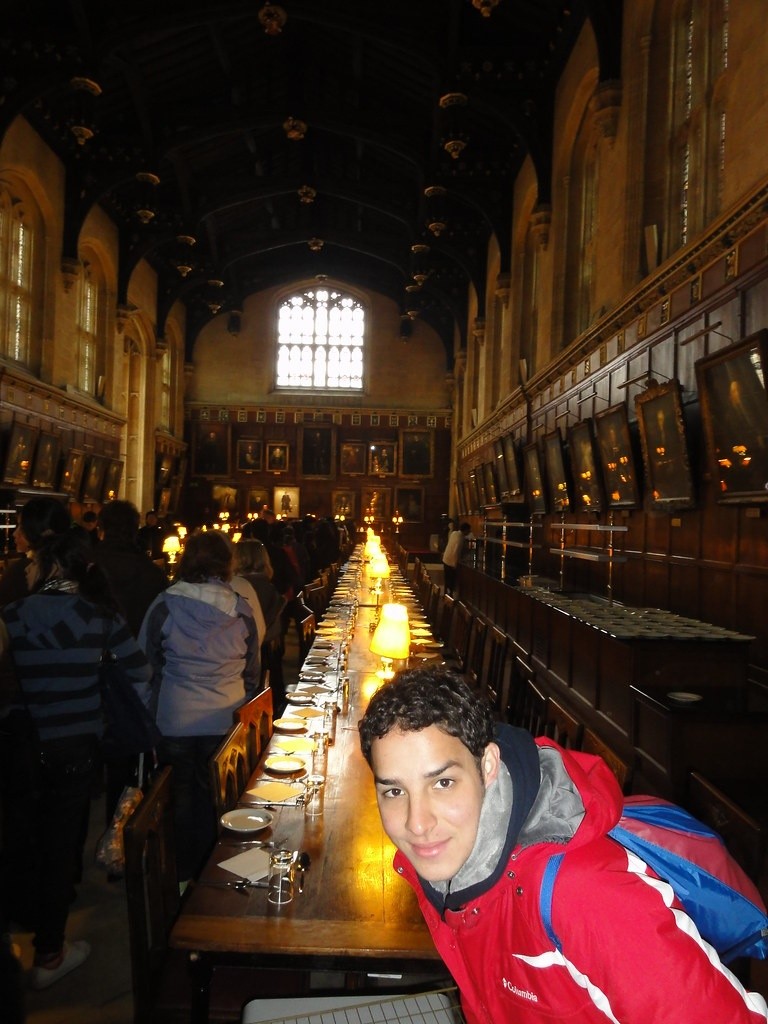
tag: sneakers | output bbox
[12,943,21,958]
[32,939,90,990]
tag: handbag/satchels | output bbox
[99,607,152,766]
[94,752,154,875]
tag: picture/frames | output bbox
[295,421,338,482]
[265,443,289,473]
[4,421,125,505]
[368,440,398,475]
[332,489,356,519]
[451,329,768,517]
[190,420,234,479]
[398,427,436,479]
[361,487,390,523]
[340,443,367,475]
[391,485,427,523]
[236,439,264,473]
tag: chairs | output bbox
[393,540,762,987]
[120,563,339,1024]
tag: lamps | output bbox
[162,513,413,686]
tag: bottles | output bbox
[273,718,307,730]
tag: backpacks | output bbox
[539,794,768,966]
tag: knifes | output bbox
[231,881,269,888]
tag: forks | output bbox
[219,838,288,848]
[200,881,251,889]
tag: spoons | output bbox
[298,852,310,894]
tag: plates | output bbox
[299,673,322,681]
[284,540,445,664]
[514,586,758,640]
[285,693,315,703]
[667,692,702,702]
[220,809,274,833]
[264,757,306,773]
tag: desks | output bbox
[168,538,452,962]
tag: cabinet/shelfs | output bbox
[549,513,630,610]
[483,514,544,584]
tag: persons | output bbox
[357,665,768,1024]
[440,518,471,596]
[0,497,353,1024]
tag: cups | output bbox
[268,542,416,905]
